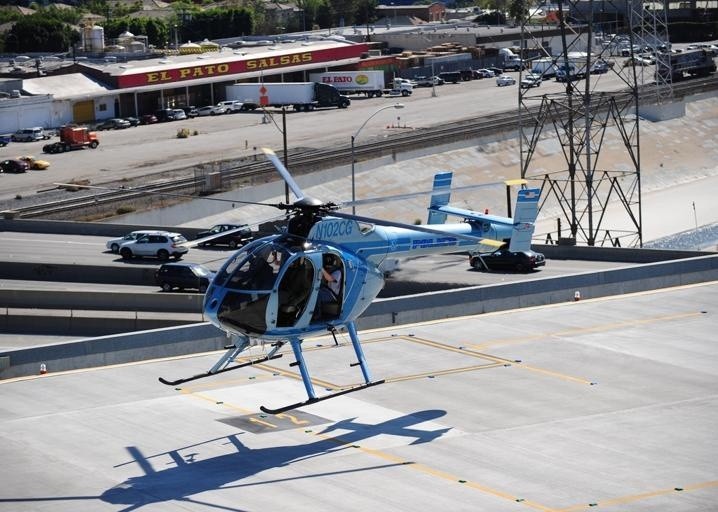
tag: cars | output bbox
[98,100,245,131]
[521,75,542,88]
[496,76,516,86]
[406,65,504,86]
[106,228,188,259]
[156,262,215,291]
[196,221,249,247]
[470,248,545,271]
[0,154,50,174]
[601,32,718,65]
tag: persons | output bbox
[296,257,343,319]
[230,249,274,297]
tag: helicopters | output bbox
[55,148,539,416]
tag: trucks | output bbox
[225,82,351,111]
[309,69,413,97]
[653,50,716,80]
[530,51,611,80]
[43,125,99,154]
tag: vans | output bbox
[0,126,44,146]
